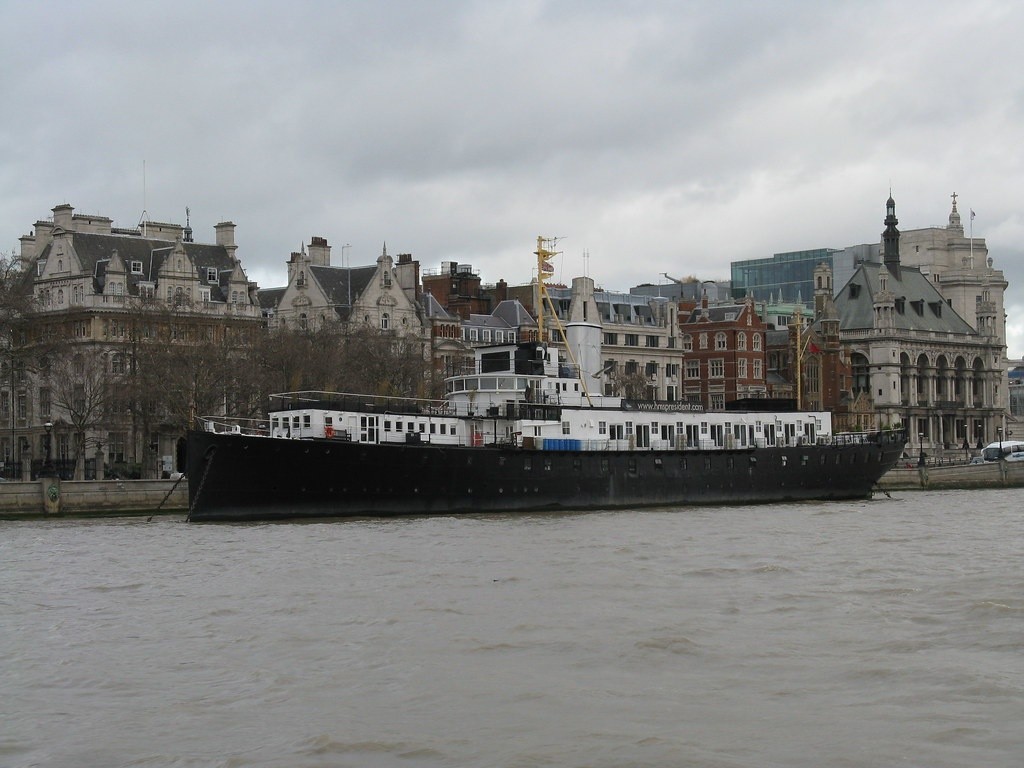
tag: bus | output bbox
[981,441,1024,464]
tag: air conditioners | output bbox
[626,434,637,450]
[818,437,830,446]
[798,435,808,447]
[776,438,786,448]
[676,435,686,450]
[724,434,735,450]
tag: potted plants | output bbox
[467,389,476,416]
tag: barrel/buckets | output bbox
[523,436,582,451]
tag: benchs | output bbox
[326,429,352,442]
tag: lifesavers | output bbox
[325,426,334,438]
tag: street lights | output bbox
[919,430,925,452]
[976,424,984,449]
[998,426,1006,459]
[43,419,55,478]
[962,422,970,449]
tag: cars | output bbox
[970,457,984,465]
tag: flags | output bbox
[808,340,820,352]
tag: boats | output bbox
[175,235,911,524]
